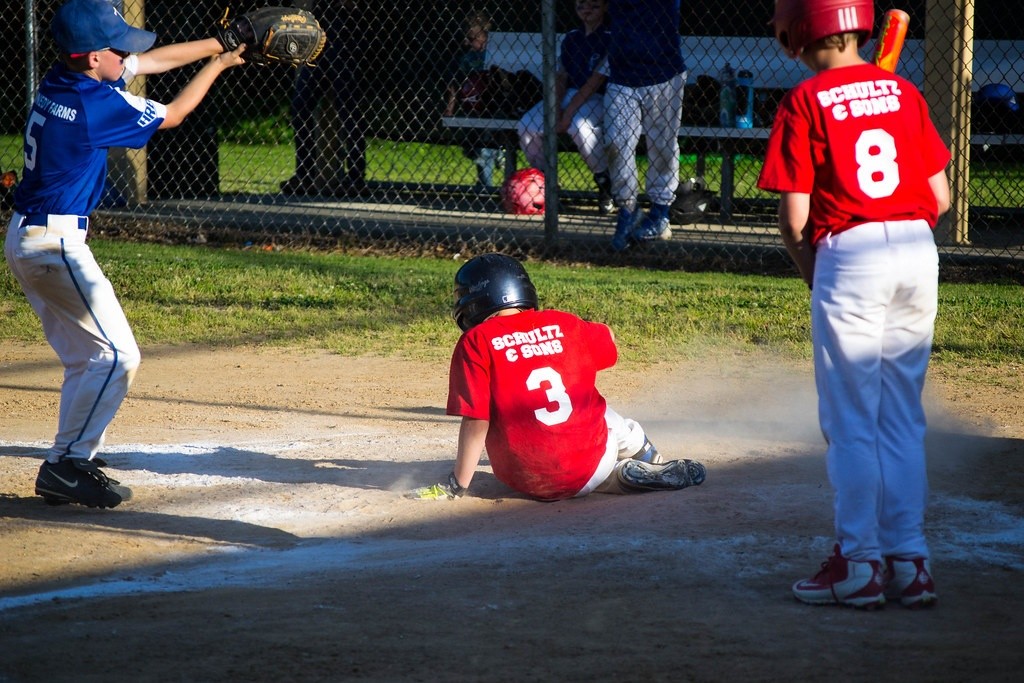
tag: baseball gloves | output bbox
[214,6,328,66]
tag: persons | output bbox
[754,0,950,607]
[3,0,326,509]
[402,252,705,503]
[433,1,687,252]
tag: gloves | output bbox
[403,473,466,502]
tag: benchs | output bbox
[441,31,1024,226]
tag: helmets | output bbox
[452,253,538,332]
[768,0,875,59]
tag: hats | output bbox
[51,0,157,58]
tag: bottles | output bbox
[718,62,754,128]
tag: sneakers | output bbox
[793,550,884,613]
[612,210,638,250]
[36,459,134,509]
[594,173,614,214]
[618,459,706,490]
[632,215,673,243]
[884,553,938,611]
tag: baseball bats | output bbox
[875,7,912,73]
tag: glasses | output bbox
[110,47,129,59]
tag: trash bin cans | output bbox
[973,84,1021,162]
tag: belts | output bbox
[21,215,88,230]
